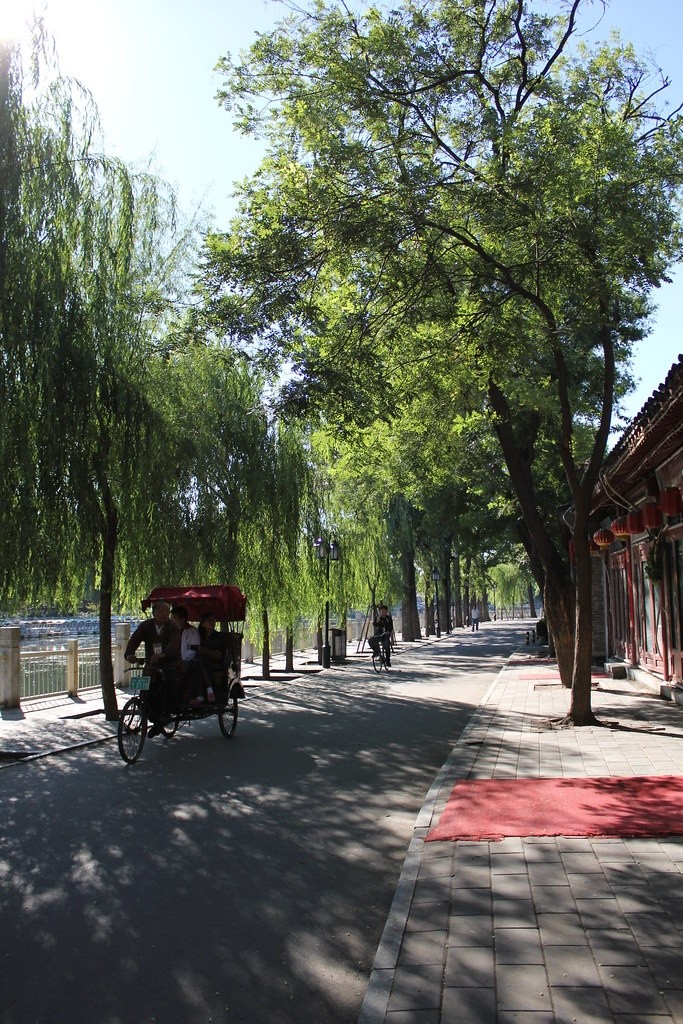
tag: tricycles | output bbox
[117,585,247,763]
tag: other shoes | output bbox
[208,692,215,702]
[386,659,391,667]
[148,726,161,739]
[190,698,205,705]
[157,709,169,720]
[372,651,381,657]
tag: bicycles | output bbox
[369,641,391,673]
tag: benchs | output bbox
[214,632,242,689]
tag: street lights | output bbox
[313,531,340,668]
[431,564,441,637]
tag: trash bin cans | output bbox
[328,628,347,662]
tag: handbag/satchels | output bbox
[183,653,214,683]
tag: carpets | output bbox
[425,775,683,842]
[507,660,556,665]
[519,672,610,680]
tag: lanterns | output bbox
[661,486,682,517]
[587,538,598,556]
[593,529,614,550]
[642,502,661,528]
[611,518,630,541]
[626,513,644,534]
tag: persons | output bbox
[471,605,479,632]
[368,605,393,667]
[125,603,227,739]
